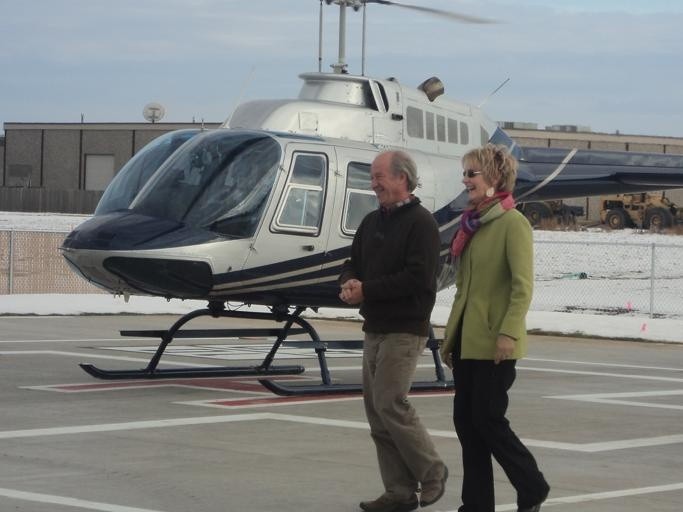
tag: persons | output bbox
[440,141,551,511]
[337,149,449,511]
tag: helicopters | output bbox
[57,0,683,400]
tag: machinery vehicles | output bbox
[512,198,586,229]
[598,190,683,232]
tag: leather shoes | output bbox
[361,494,418,511]
[421,467,448,506]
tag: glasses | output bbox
[463,169,483,178]
